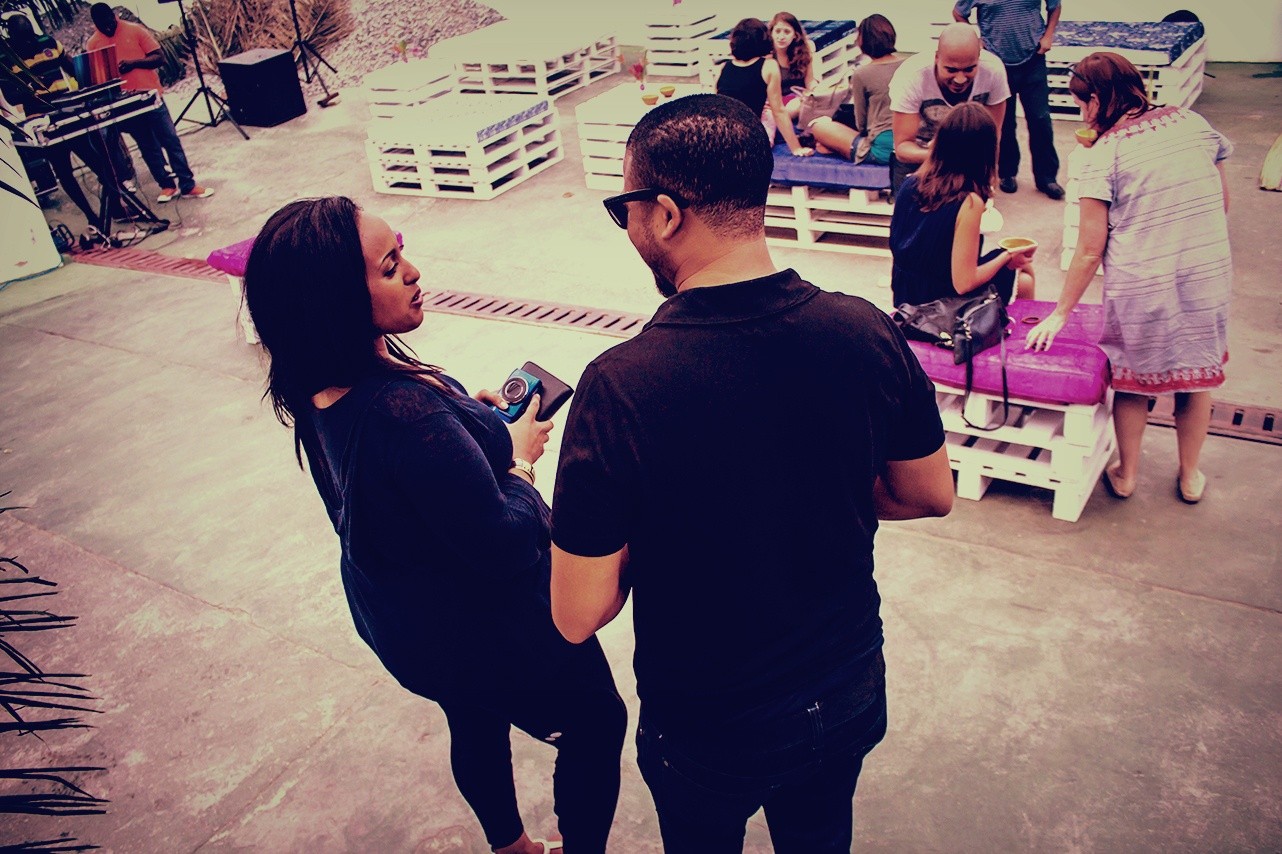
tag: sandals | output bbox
[533,838,562,854]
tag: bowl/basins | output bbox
[997,238,1038,258]
[660,86,675,97]
[641,95,658,105]
[1074,128,1098,147]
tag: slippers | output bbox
[1103,458,1134,499]
[1179,467,1206,503]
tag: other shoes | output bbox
[182,187,215,199]
[87,226,100,238]
[1000,177,1018,193]
[123,179,136,192]
[157,187,180,202]
[114,206,139,222]
[39,199,59,209]
[1036,180,1065,198]
[816,142,834,154]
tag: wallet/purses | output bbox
[490,359,573,424]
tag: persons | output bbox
[811,14,907,165]
[651,0,1232,506]
[87,5,214,202]
[236,192,627,854]
[0,14,139,232]
[551,95,958,854]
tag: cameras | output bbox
[489,367,541,423]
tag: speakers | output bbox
[217,49,306,127]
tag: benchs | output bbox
[700,19,862,96]
[367,92,566,200]
[763,121,898,256]
[1017,21,1204,121]
[429,17,621,99]
[887,300,1117,523]
[207,232,403,345]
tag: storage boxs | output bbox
[10,89,163,147]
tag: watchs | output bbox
[509,458,536,485]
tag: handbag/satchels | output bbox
[789,83,853,131]
[893,292,1013,366]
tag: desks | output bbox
[1061,143,1107,274]
[366,57,460,137]
[576,81,700,190]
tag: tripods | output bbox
[158,0,250,140]
[287,0,337,95]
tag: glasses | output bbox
[1065,64,1096,93]
[603,185,690,230]
